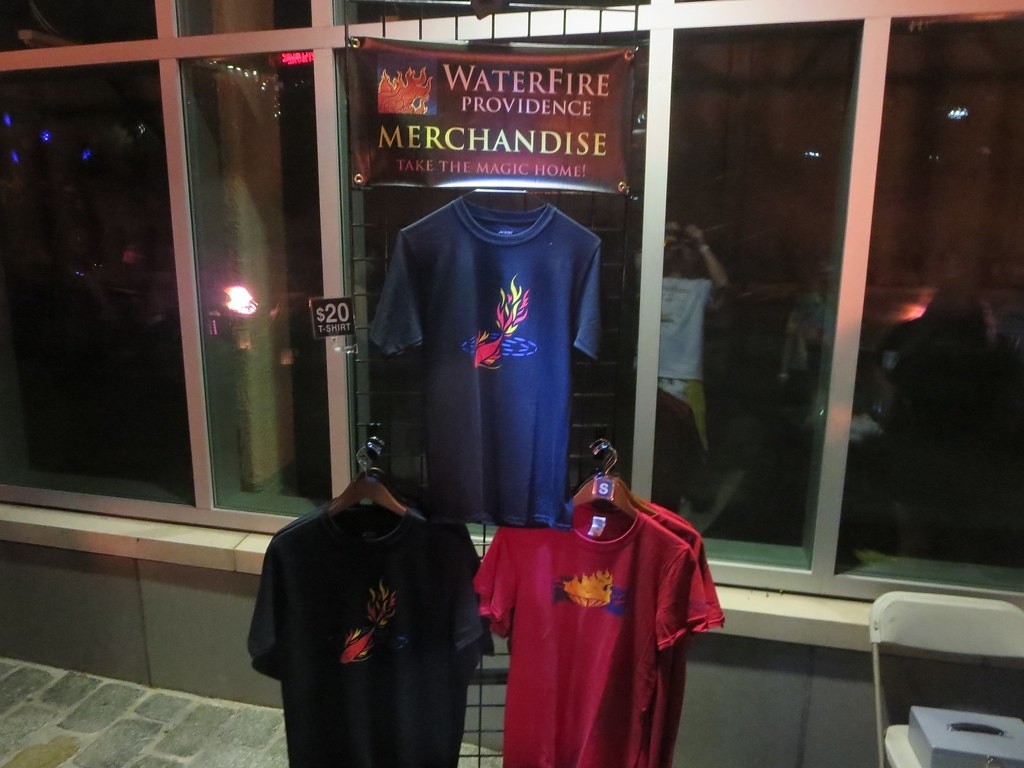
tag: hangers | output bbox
[463,189,547,211]
[329,435,406,520]
[572,439,658,523]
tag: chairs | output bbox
[869,591,1024,768]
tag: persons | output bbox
[796,267,999,446]
[624,223,727,451]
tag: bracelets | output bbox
[700,245,709,252]
